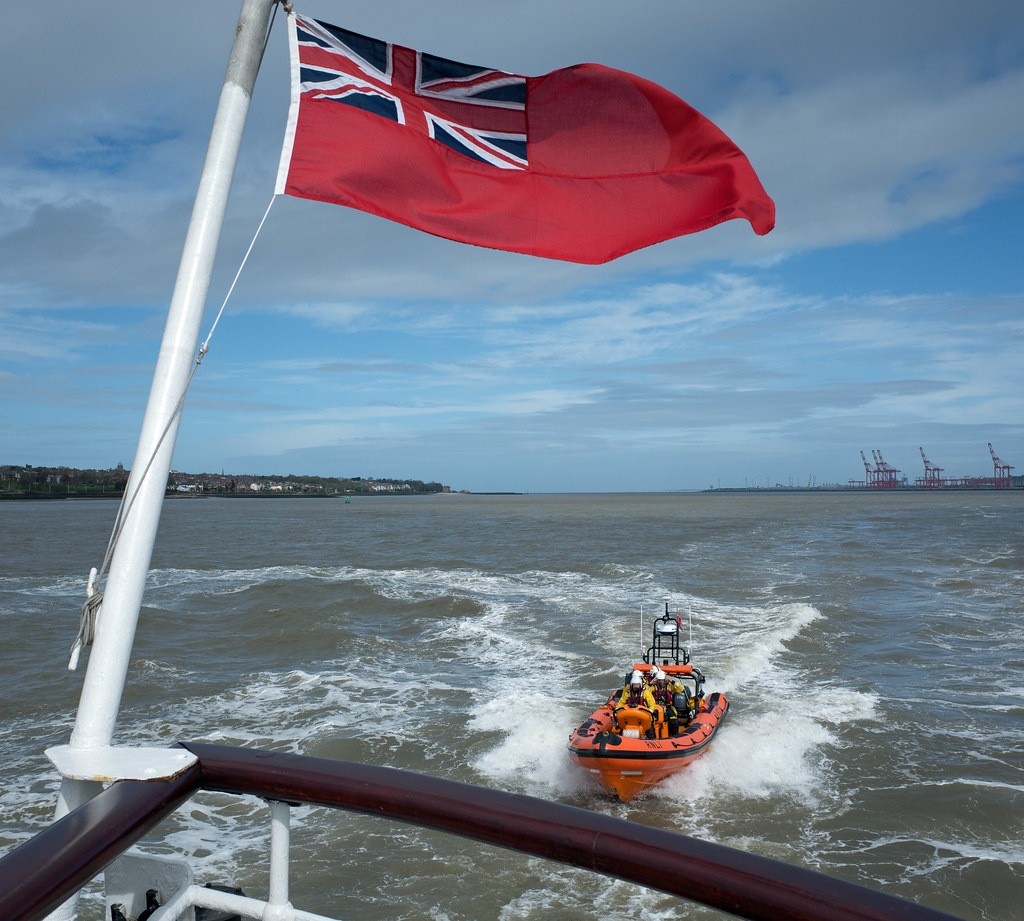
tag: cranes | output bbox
[919,446,944,487]
[860,449,901,489]
[988,442,1015,487]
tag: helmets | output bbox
[656,671,666,679]
[631,676,643,688]
[650,666,661,677]
[632,670,644,678]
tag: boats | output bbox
[565,595,730,804]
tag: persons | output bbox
[614,676,655,740]
[632,665,662,693]
[647,671,684,716]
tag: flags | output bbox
[271,12,775,264]
[677,610,682,630]
